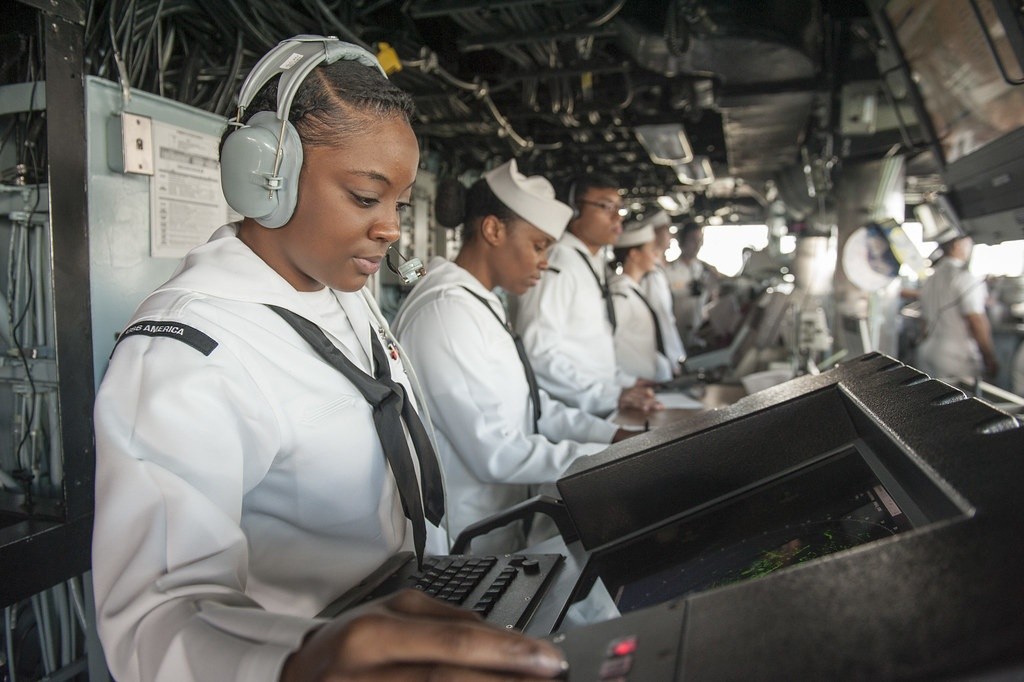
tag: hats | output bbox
[927,247,946,267]
[483,157,574,243]
[610,220,655,248]
[638,207,671,229]
[935,224,973,245]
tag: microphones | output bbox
[383,245,427,284]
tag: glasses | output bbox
[574,196,628,216]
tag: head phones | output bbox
[568,173,583,219]
[222,32,388,228]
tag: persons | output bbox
[515,171,663,416]
[913,217,1000,383]
[91,34,570,682]
[390,157,649,555]
[609,198,721,384]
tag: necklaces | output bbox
[376,325,400,361]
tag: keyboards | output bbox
[303,549,564,630]
